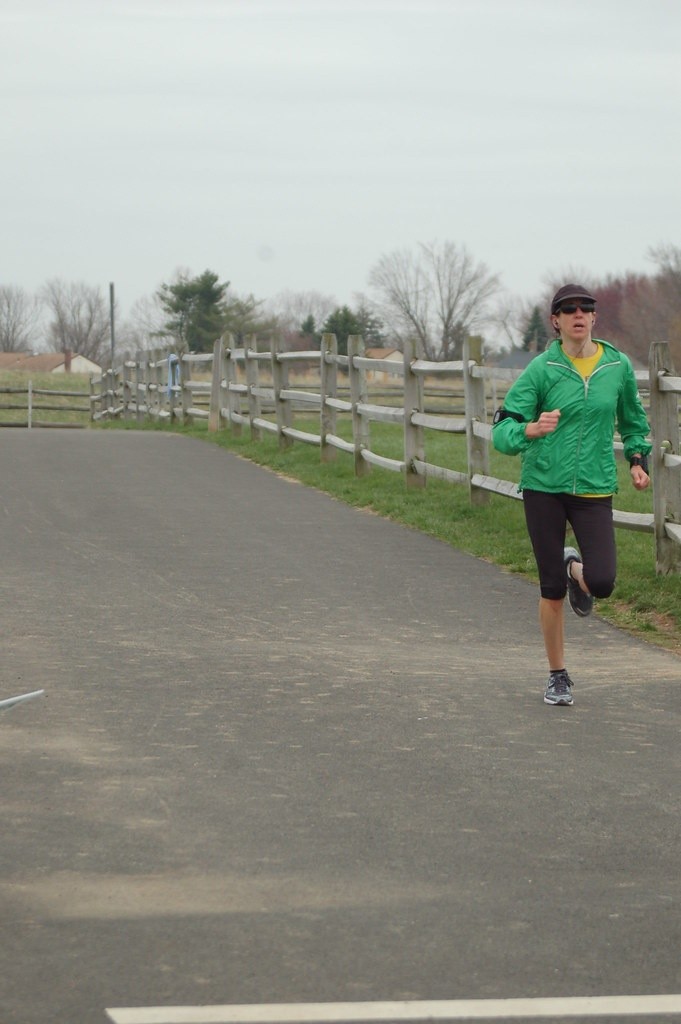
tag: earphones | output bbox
[592,320,594,324]
[554,320,558,325]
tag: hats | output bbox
[551,284,597,313]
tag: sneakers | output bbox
[544,670,573,706]
[564,547,593,617]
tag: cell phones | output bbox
[643,455,649,474]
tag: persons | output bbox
[492,281,652,707]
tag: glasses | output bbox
[552,302,594,314]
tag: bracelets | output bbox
[629,455,644,469]
